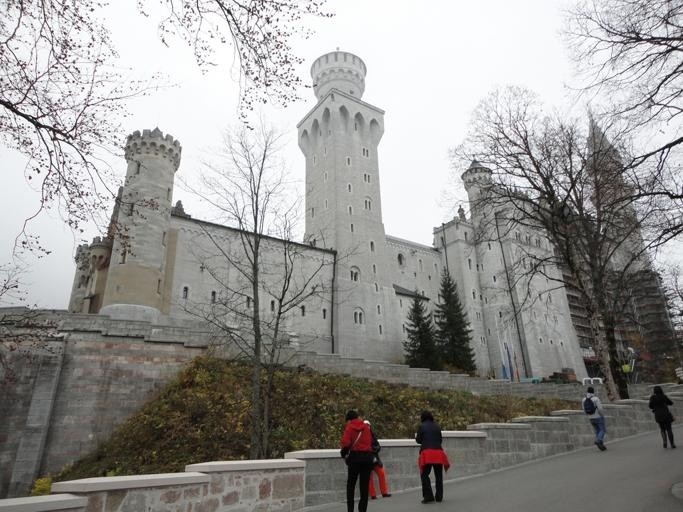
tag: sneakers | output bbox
[421,498,443,504]
[381,494,391,498]
[371,495,378,500]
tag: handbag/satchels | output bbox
[340,447,352,461]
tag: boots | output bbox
[661,432,676,449]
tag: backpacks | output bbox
[584,395,597,415]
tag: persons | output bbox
[626,346,640,364]
[362,420,392,500]
[649,385,676,450]
[338,409,375,512]
[415,409,451,503]
[581,386,609,452]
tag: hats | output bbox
[363,419,371,426]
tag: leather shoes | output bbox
[594,440,607,451]
